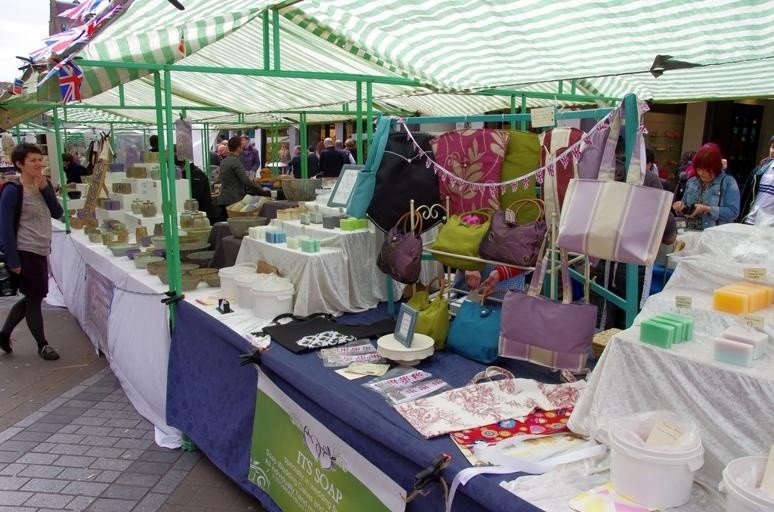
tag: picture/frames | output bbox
[327,164,365,208]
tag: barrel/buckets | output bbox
[218,262,258,296]
[251,281,297,319]
[234,273,271,308]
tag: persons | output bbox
[174,134,357,226]
[0,141,64,360]
[739,134,774,229]
[149,135,159,151]
[611,134,741,243]
[59,141,97,199]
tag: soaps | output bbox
[711,281,774,315]
[714,327,770,367]
[639,312,695,346]
[248,206,369,252]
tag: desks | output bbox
[161,287,774,512]
[45,214,217,453]
[234,197,442,316]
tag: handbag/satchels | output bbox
[0,262,17,297]
[429,206,490,275]
[496,285,600,373]
[444,288,509,368]
[369,210,428,285]
[403,274,448,351]
[483,198,544,269]
[552,93,678,269]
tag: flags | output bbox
[56,58,84,104]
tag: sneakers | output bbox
[36,341,61,361]
[0,330,14,354]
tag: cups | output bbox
[66,148,181,243]
[270,191,277,201]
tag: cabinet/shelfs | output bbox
[568,224,774,486]
[95,176,189,240]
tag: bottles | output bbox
[180,198,210,229]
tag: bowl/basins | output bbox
[280,178,324,202]
[106,236,219,289]
[228,216,266,238]
[226,197,274,218]
[217,265,294,319]
